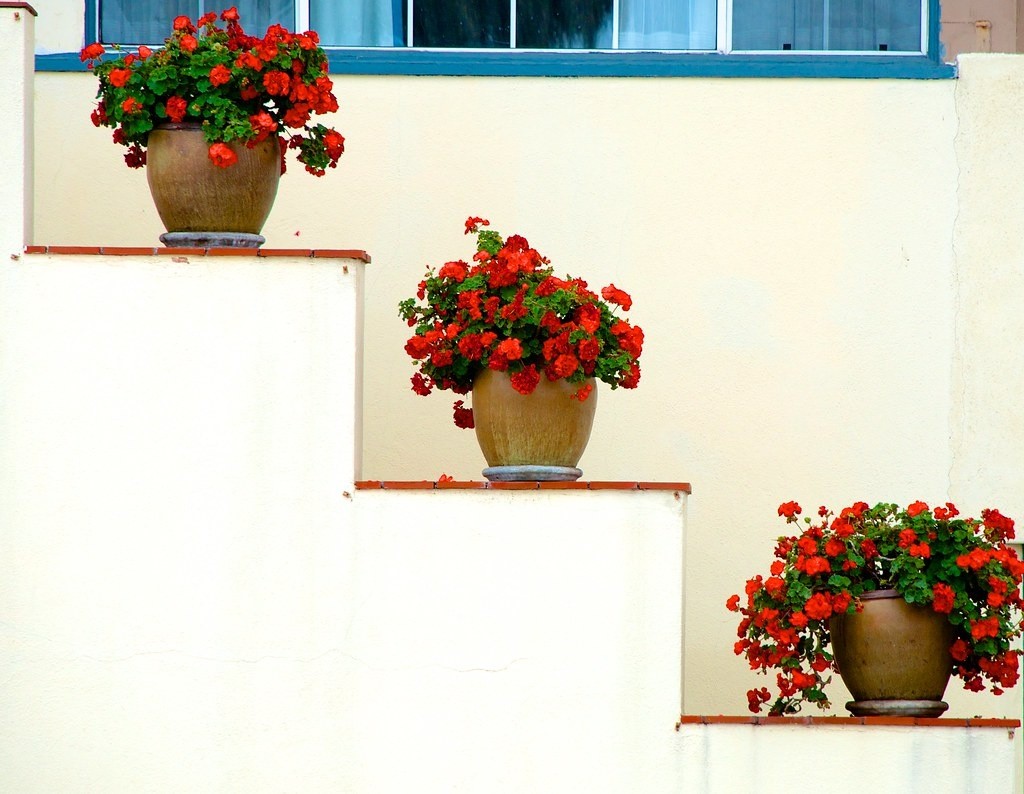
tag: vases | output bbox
[147,114,281,248]
[471,362,598,481]
[829,589,958,717]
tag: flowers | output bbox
[724,500,1024,717]
[78,7,345,178]
[397,214,645,430]
